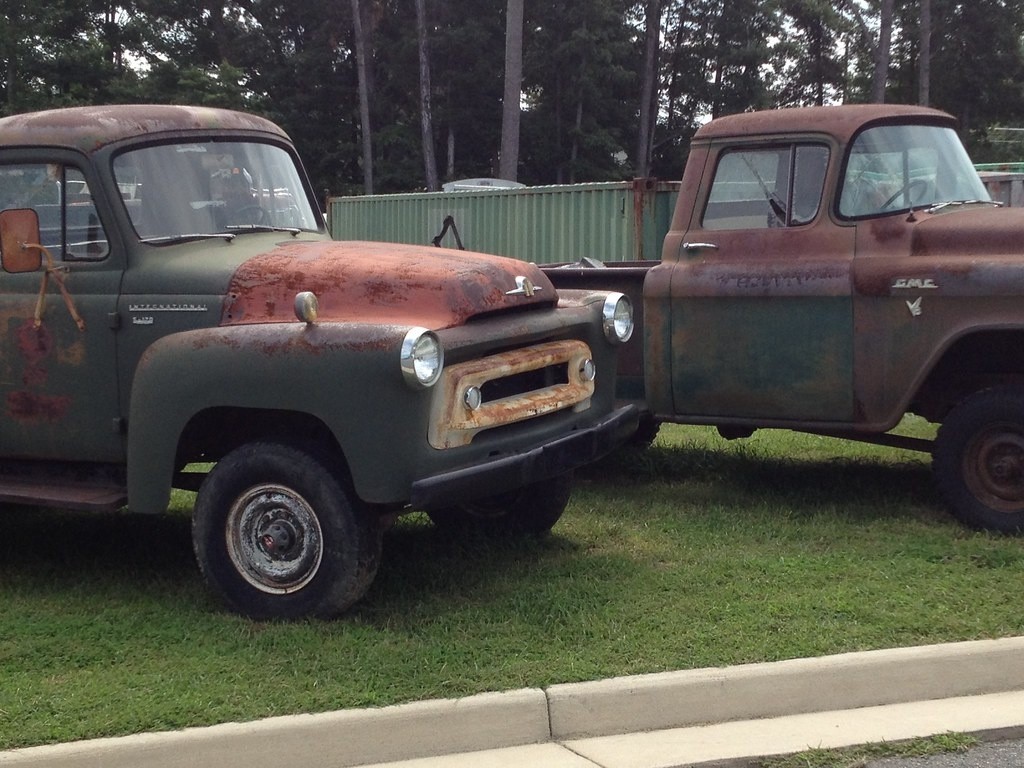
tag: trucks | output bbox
[0,105,637,622]
[324,102,1024,537]
